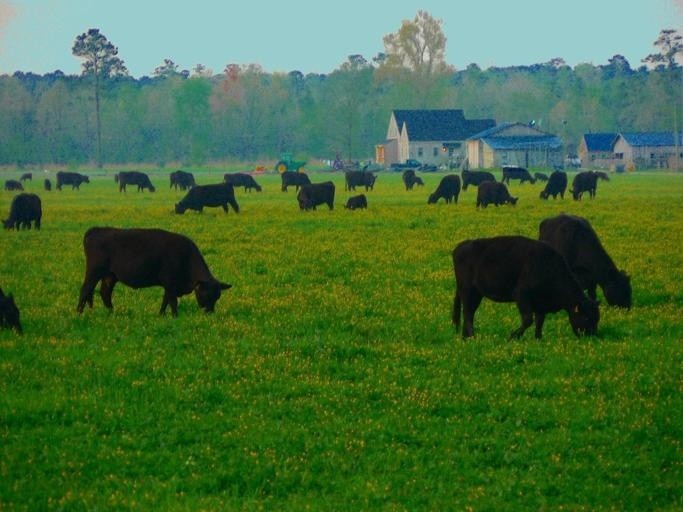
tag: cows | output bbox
[534,172,549,182]
[0,193,43,232]
[501,166,537,186]
[223,173,262,194]
[77,226,232,320]
[176,170,197,191]
[569,170,597,201]
[5,180,25,192]
[594,171,610,181]
[476,181,519,209]
[538,212,632,315]
[174,182,240,215]
[56,171,90,192]
[461,169,497,191]
[451,234,603,343]
[539,170,568,200]
[402,169,424,191]
[45,179,52,190]
[345,171,378,192]
[297,181,336,211]
[119,171,156,193]
[427,174,461,205]
[343,194,368,210]
[0,286,24,340]
[114,174,118,183]
[281,171,311,193]
[169,172,190,191]
[19,172,32,183]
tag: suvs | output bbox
[566,155,581,168]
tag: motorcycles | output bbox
[418,164,437,172]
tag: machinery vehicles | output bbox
[256,153,307,174]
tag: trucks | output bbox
[391,159,423,173]
